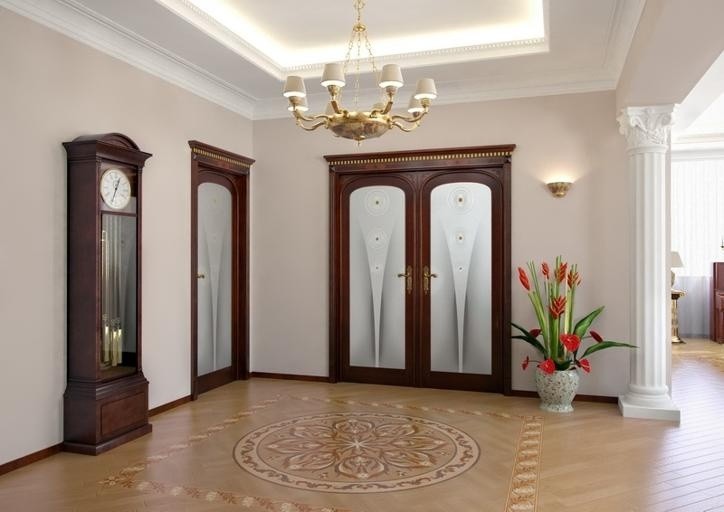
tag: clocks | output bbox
[56,129,153,456]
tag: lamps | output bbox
[669,251,683,292]
[282,0,438,141]
[546,181,574,199]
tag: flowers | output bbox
[510,255,640,374]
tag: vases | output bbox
[535,364,581,414]
